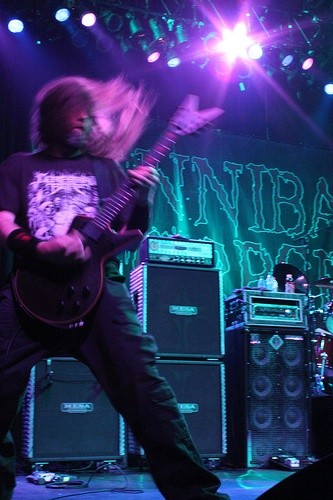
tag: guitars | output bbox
[12,95,224,325]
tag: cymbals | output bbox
[273,262,309,295]
[314,277,333,287]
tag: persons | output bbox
[0,78,234,500]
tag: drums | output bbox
[312,332,333,377]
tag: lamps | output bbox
[72,0,321,72]
